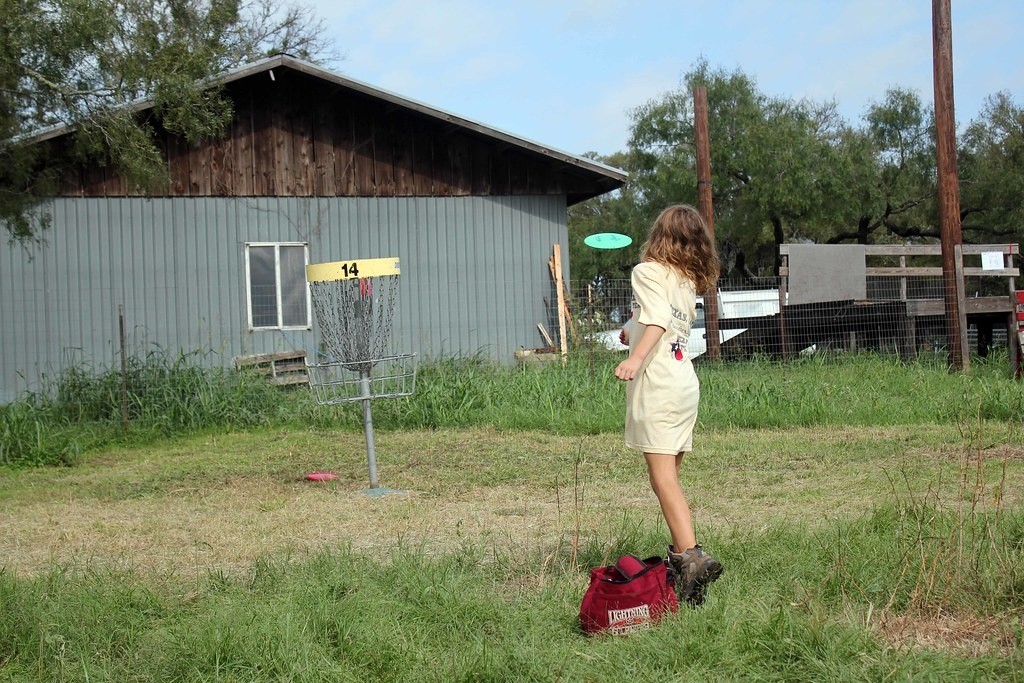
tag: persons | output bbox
[614,203,724,609]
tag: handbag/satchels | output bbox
[580,554,679,638]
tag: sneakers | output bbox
[664,556,681,584]
[667,545,723,610]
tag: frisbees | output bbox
[584,233,634,249]
